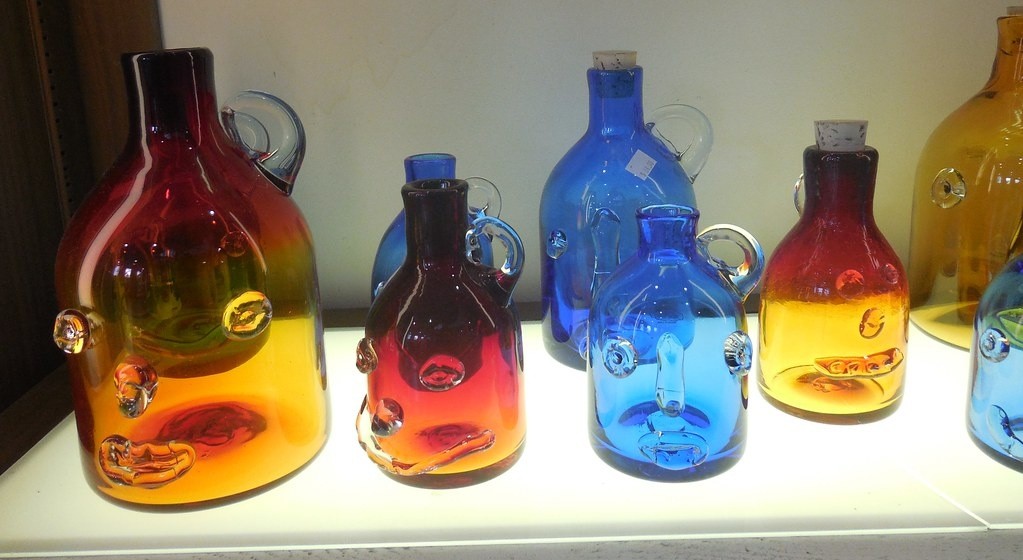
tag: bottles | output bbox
[371,153,502,305]
[908,7,1023,350]
[759,121,910,414]
[355,179,525,476]
[52,48,333,505]
[541,51,714,360]
[969,254,1023,463]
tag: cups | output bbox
[588,205,765,470]
